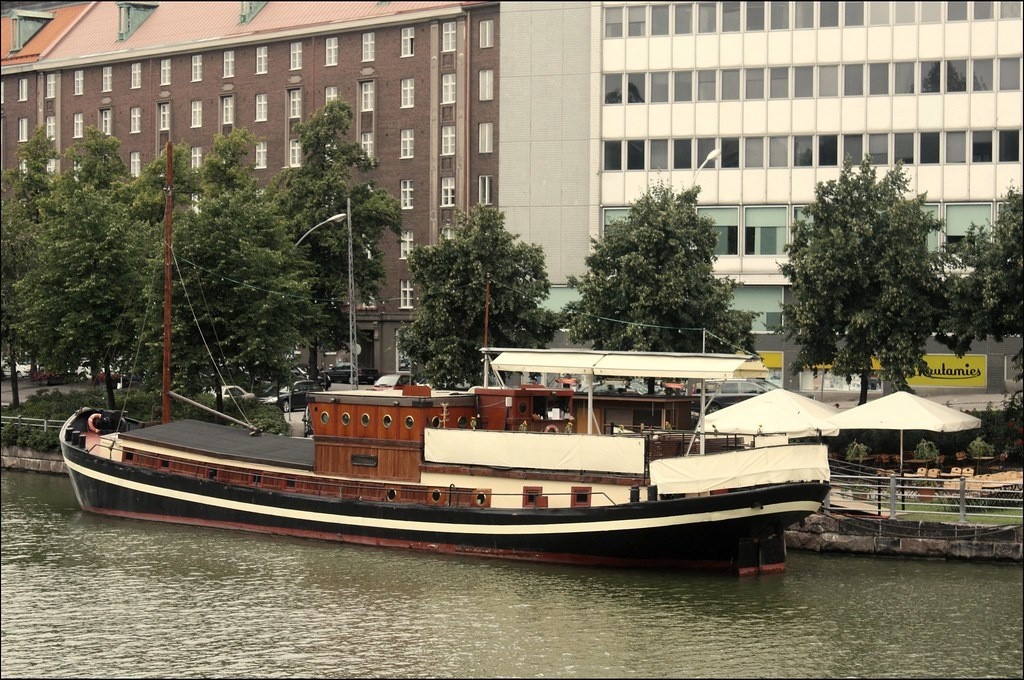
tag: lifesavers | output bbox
[544,425,559,433]
[87,413,102,433]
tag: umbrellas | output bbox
[698,389,981,470]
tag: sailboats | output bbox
[59,140,833,574]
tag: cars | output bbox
[322,362,380,385]
[67,361,120,381]
[204,385,254,399]
[579,381,647,395]
[679,379,815,415]
[374,373,415,388]
[258,379,325,412]
[1,357,39,378]
[289,363,331,391]
